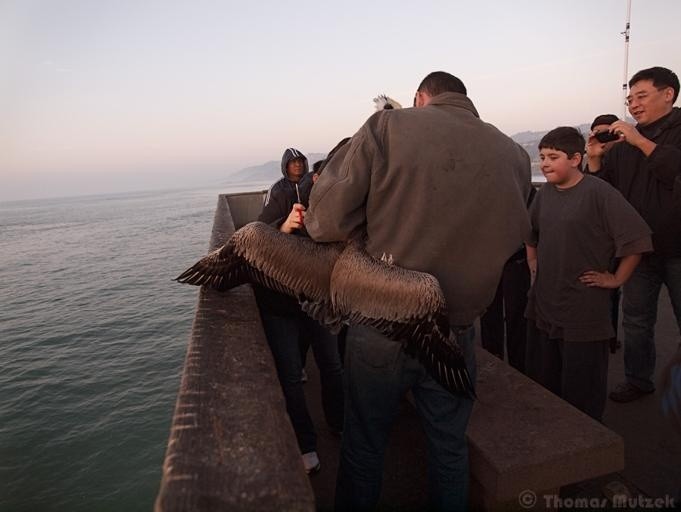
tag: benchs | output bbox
[466,343,626,509]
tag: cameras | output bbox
[595,127,621,144]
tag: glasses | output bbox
[624,87,662,106]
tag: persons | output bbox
[581,114,627,353]
[584,65,681,404]
[249,125,658,479]
[300,70,534,511]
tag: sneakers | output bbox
[608,383,648,403]
[303,452,319,474]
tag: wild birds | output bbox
[168,93,480,404]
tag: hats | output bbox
[591,114,619,129]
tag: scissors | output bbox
[295,183,304,228]
